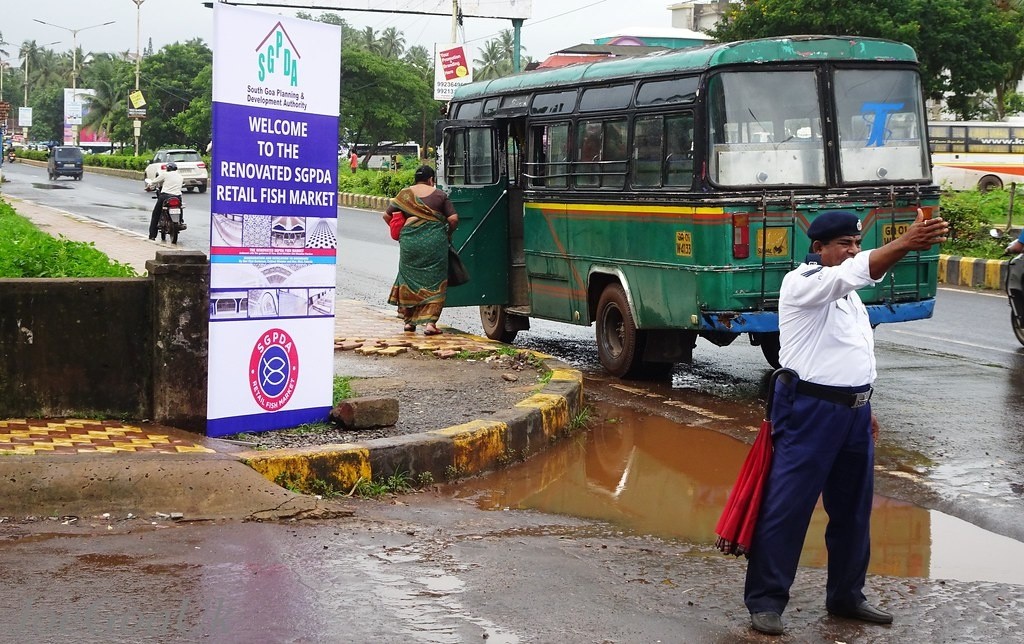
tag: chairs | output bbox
[452,149,693,186]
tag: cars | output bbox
[23,141,60,153]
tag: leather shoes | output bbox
[839,599,893,624]
[751,611,783,636]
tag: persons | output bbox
[350,149,358,173]
[383,165,458,335]
[744,208,949,633]
[147,163,184,241]
[7,144,15,156]
[1004,227,1024,253]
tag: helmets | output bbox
[166,163,177,171]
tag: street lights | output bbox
[8,41,62,144]
[32,18,116,147]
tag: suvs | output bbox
[144,149,209,194]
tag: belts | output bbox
[778,373,874,408]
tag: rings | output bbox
[925,220,927,226]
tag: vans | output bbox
[44,146,84,181]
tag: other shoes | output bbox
[424,323,443,335]
[404,323,416,332]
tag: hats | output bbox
[807,212,862,240]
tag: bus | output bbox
[909,121,1024,194]
[436,35,940,381]
[337,141,420,170]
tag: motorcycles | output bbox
[145,178,187,244]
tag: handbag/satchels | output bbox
[448,236,469,286]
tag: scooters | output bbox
[989,227,1024,347]
[8,151,16,163]
